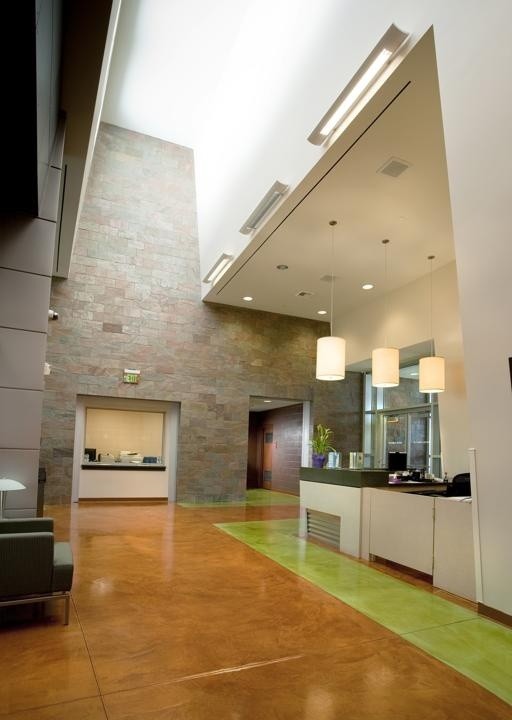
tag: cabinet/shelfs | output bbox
[296,478,477,602]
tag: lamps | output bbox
[0,478,27,517]
[315,221,448,396]
[238,178,290,237]
[307,22,409,148]
[204,252,234,285]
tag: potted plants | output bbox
[307,424,338,469]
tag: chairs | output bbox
[444,473,471,495]
[0,518,76,626]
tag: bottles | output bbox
[444,472,448,482]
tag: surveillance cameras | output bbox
[48,309,59,320]
